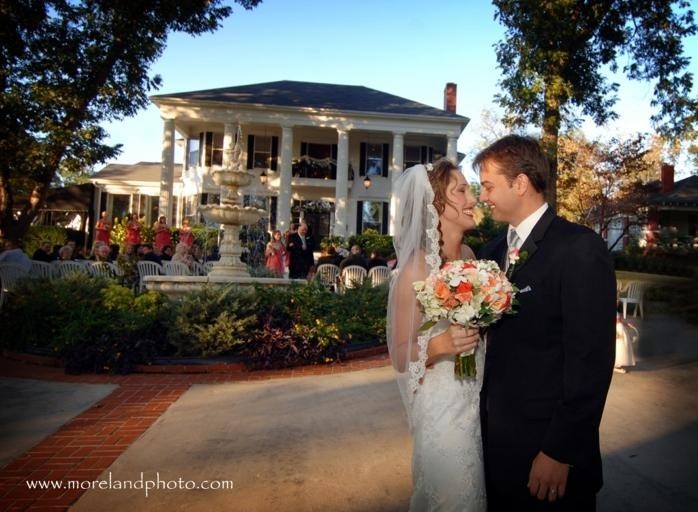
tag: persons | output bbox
[1,207,221,289]
[385,157,488,512]
[473,134,616,512]
[239,218,399,293]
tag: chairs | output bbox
[616,278,622,308]
[617,280,655,320]
[0,248,391,296]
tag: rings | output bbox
[550,489,559,496]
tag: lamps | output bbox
[260,125,267,185]
[364,132,371,189]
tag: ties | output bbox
[503,230,521,282]
